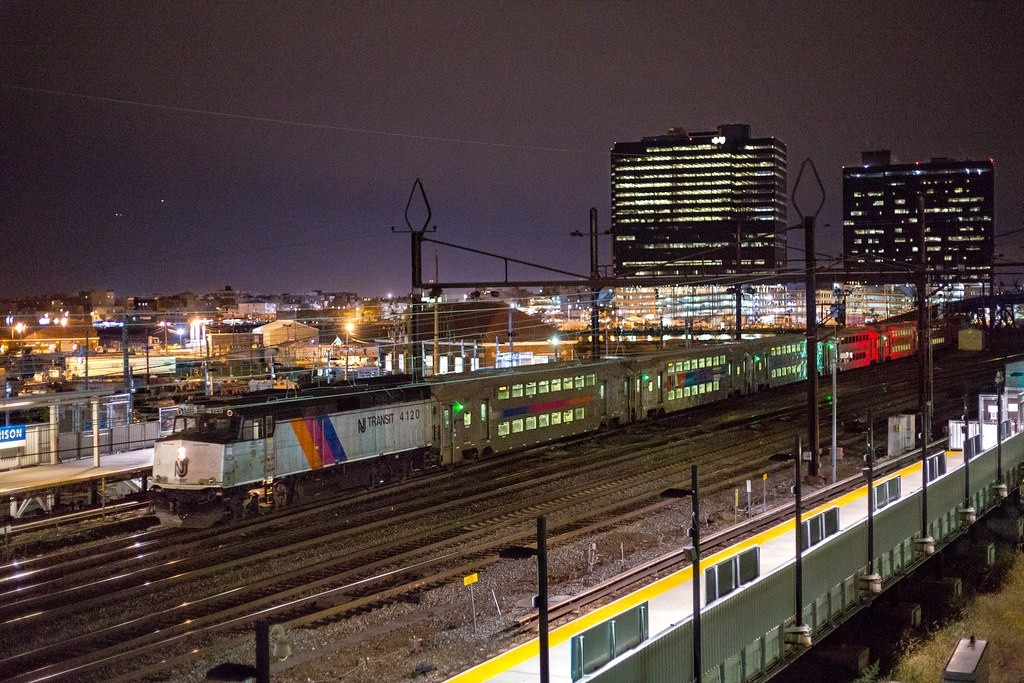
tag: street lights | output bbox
[176,329,184,345]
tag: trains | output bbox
[147,319,952,518]
